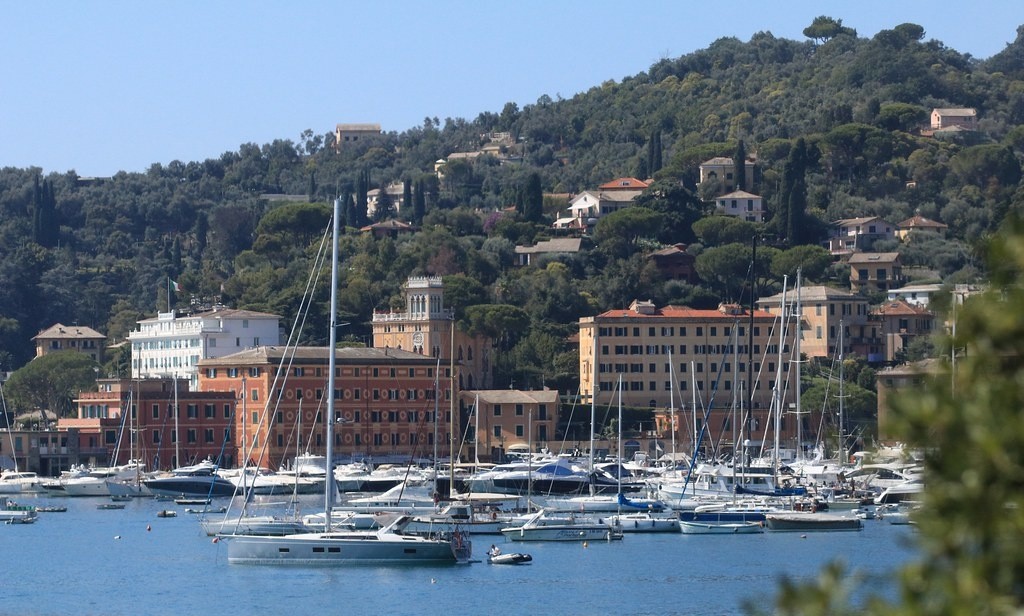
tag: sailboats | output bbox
[0,197,927,573]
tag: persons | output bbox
[490,544,501,556]
